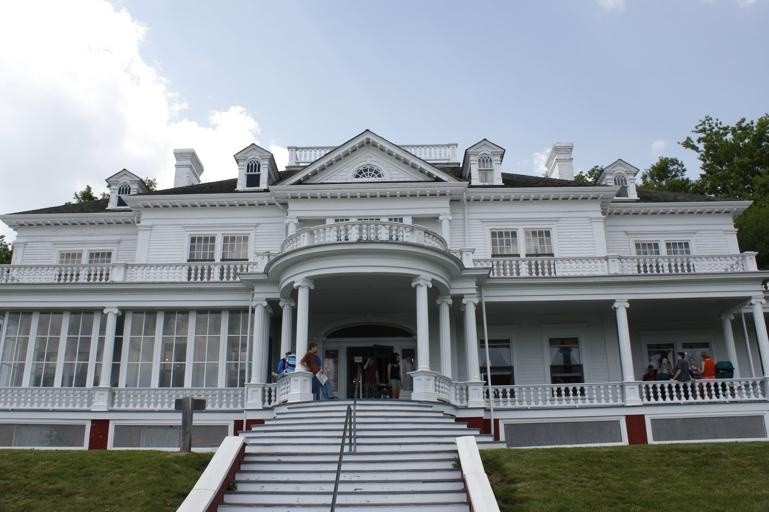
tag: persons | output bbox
[387,352,402,400]
[363,357,378,398]
[275,344,335,400]
[643,350,716,400]
